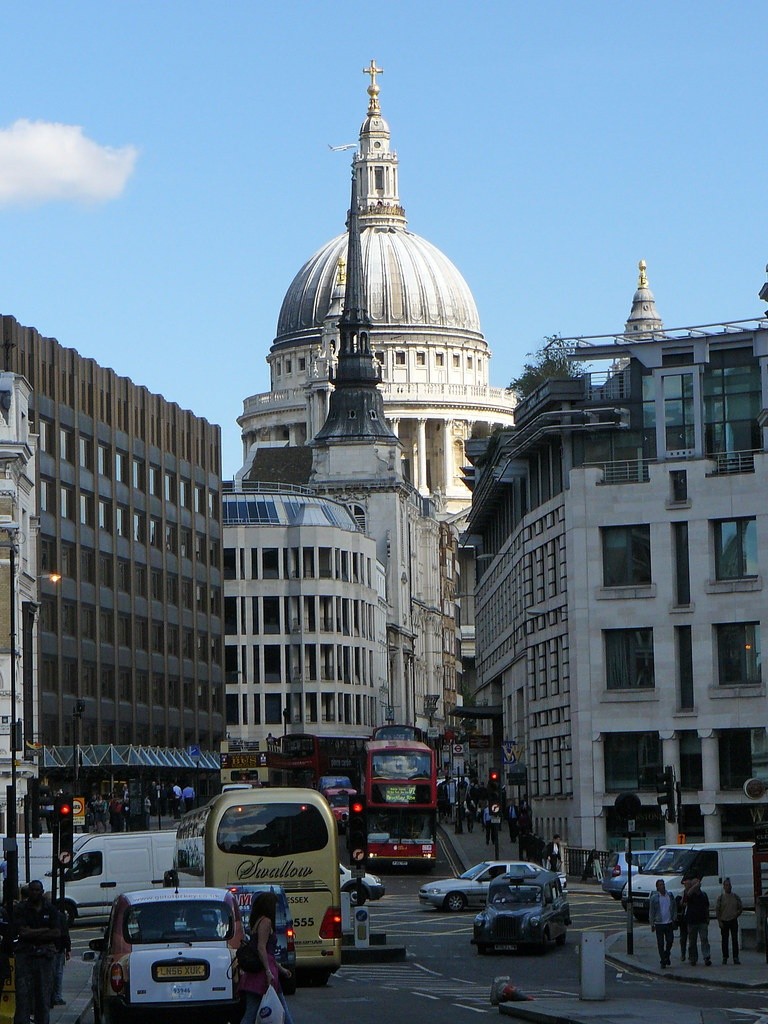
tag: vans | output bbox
[35,827,180,931]
[620,842,768,924]
[602,850,673,901]
[318,776,359,833]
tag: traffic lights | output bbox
[489,767,501,802]
[31,777,51,838]
[656,765,676,823]
[348,793,368,865]
[59,797,73,869]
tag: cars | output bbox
[338,862,387,907]
[417,860,569,914]
[469,869,571,957]
[80,886,251,1024]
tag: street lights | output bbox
[1,520,22,985]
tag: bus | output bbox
[358,740,441,876]
[171,781,343,988]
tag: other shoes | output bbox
[705,960,711,965]
[55,999,66,1005]
[734,961,741,965]
[691,962,696,966]
[722,960,727,964]
[662,962,665,968]
[681,955,685,961]
[666,958,670,964]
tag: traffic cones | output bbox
[489,976,537,1007]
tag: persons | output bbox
[172,783,181,819]
[95,795,108,833]
[46,795,54,833]
[548,835,561,875]
[677,880,692,961]
[686,878,712,966]
[87,792,97,812]
[520,794,528,816]
[51,908,72,1007]
[0,907,11,1004]
[650,879,676,968]
[507,800,518,843]
[463,797,476,833]
[13,881,61,1024]
[239,892,293,1024]
[483,805,493,845]
[150,781,156,816]
[183,784,195,812]
[716,880,743,965]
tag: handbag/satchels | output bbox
[256,984,286,1024]
[235,919,263,975]
